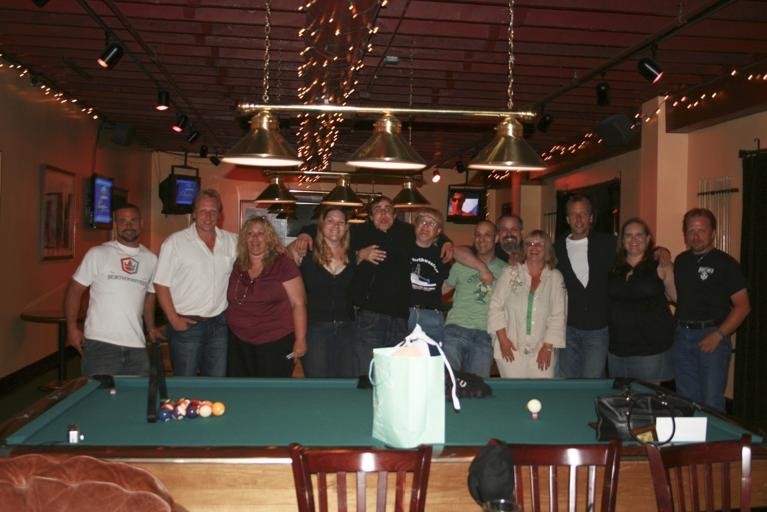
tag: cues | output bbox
[699,177,730,255]
[544,208,557,242]
[148,340,168,422]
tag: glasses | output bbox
[416,218,437,228]
[526,240,546,248]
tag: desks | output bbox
[20,283,88,394]
[0,375,767,461]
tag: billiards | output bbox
[160,397,225,422]
[527,399,542,413]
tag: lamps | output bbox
[97,33,120,68]
[254,168,431,223]
[175,114,188,129]
[220,3,304,166]
[636,46,663,87]
[595,72,612,109]
[468,1,549,171]
[187,130,220,166]
[345,110,427,169]
[156,88,171,111]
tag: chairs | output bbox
[645,432,753,512]
[487,438,617,512]
[289,442,432,512]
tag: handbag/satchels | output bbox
[592,393,702,445]
[372,345,447,449]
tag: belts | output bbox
[178,313,221,323]
[410,303,441,312]
[675,319,719,330]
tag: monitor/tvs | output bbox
[446,184,487,224]
[90,175,113,231]
[158,175,201,214]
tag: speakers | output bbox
[111,123,136,146]
[595,115,635,145]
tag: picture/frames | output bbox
[39,163,76,260]
